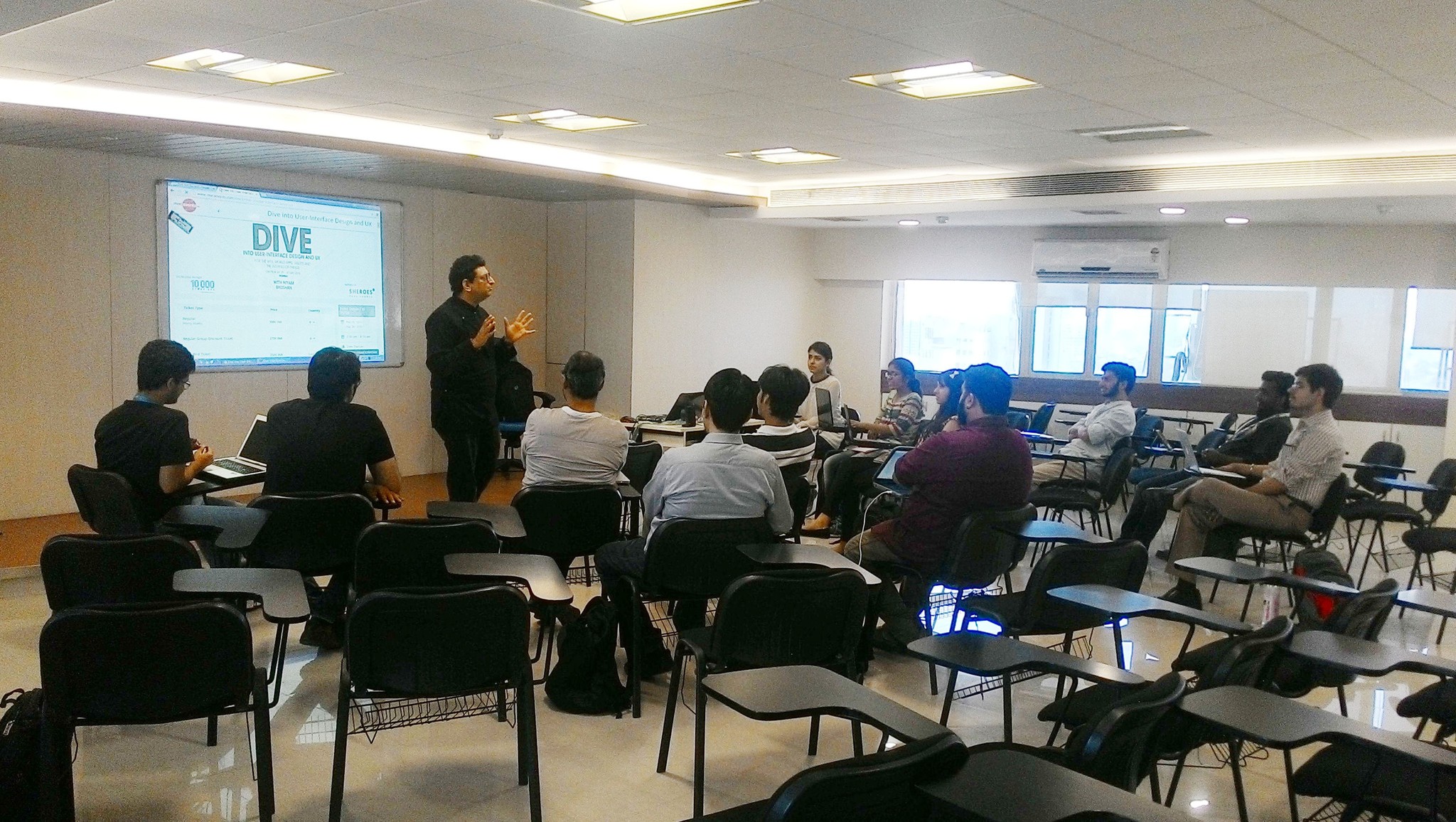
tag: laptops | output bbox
[636,392,706,422]
[815,387,857,429]
[1156,429,1197,453]
[872,447,916,497]
[1175,429,1246,480]
[844,402,891,446]
[199,414,268,481]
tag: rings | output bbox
[1204,454,1207,458]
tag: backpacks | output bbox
[495,360,537,422]
[1290,544,1359,629]
[544,594,632,719]
[0,688,79,822]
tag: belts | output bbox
[1286,495,1318,516]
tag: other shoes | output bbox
[800,527,831,539]
[299,615,346,650]
[623,648,676,678]
[527,596,581,628]
[1156,586,1203,610]
[1144,487,1181,513]
[871,625,904,655]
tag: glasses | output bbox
[475,272,494,284]
[182,381,191,390]
[1291,383,1312,390]
[884,371,901,379]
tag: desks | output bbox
[736,544,882,585]
[1276,630,1456,678]
[913,750,1199,822]
[1392,589,1456,619]
[172,568,311,624]
[616,419,766,534]
[1046,584,1251,636]
[701,665,949,743]
[995,520,1112,544]
[1175,685,1456,772]
[1342,462,1415,473]
[1145,446,1181,457]
[444,553,574,604]
[906,629,1146,692]
[426,501,526,539]
[1055,419,1077,425]
[1174,557,1359,595]
[1059,410,1089,417]
[1159,417,1214,424]
[1373,477,1456,495]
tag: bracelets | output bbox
[1248,463,1255,476]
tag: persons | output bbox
[741,364,816,480]
[794,341,847,514]
[801,358,966,554]
[521,350,629,624]
[1031,362,1137,501]
[95,339,248,569]
[1115,371,1295,551]
[594,369,793,676]
[1153,363,1346,611]
[843,363,1034,653]
[262,346,405,649]
[425,254,537,503]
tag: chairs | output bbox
[39,362,1456,822]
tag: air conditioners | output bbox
[1031,239,1170,281]
